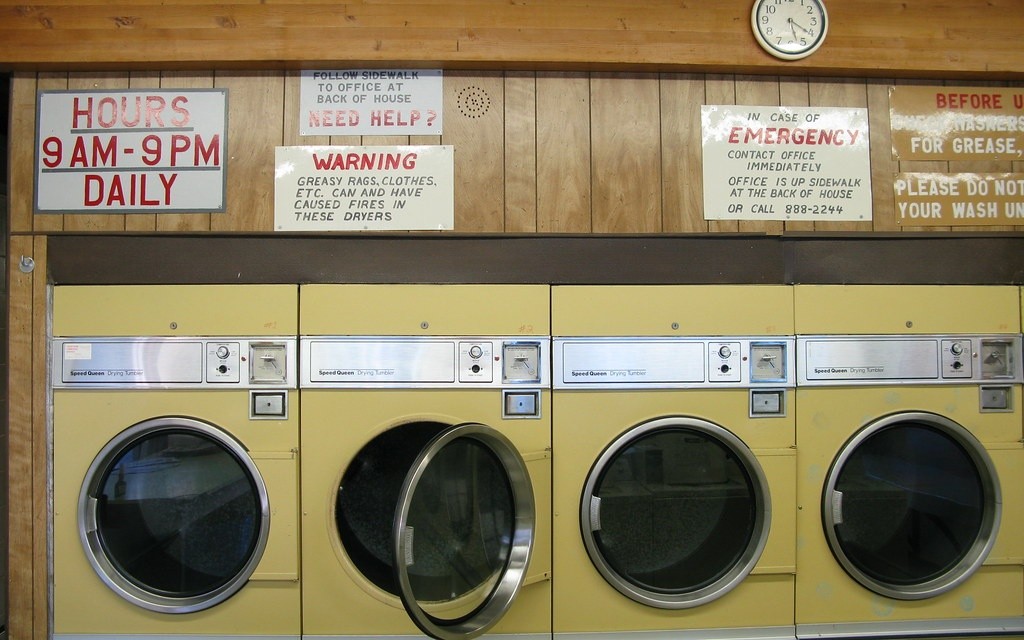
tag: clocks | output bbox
[750,0,828,61]
